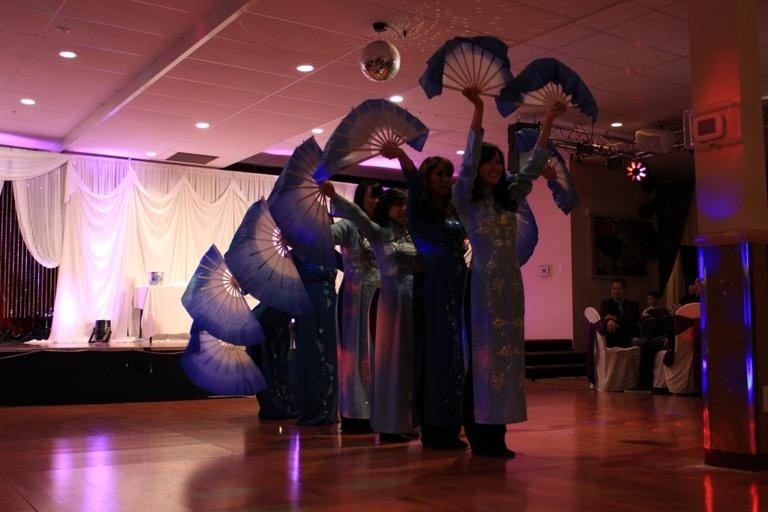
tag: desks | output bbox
[134,285,189,344]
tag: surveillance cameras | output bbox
[607,155,621,170]
[624,159,650,186]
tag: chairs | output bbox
[583,302,702,397]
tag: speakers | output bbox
[506,121,541,175]
[634,128,674,155]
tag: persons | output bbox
[239,177,434,445]
[638,290,675,351]
[589,276,640,390]
[449,87,567,457]
[377,138,559,450]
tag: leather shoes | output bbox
[405,431,421,439]
[379,433,410,442]
[471,446,515,459]
[449,438,468,449]
[423,441,451,450]
[623,386,652,394]
